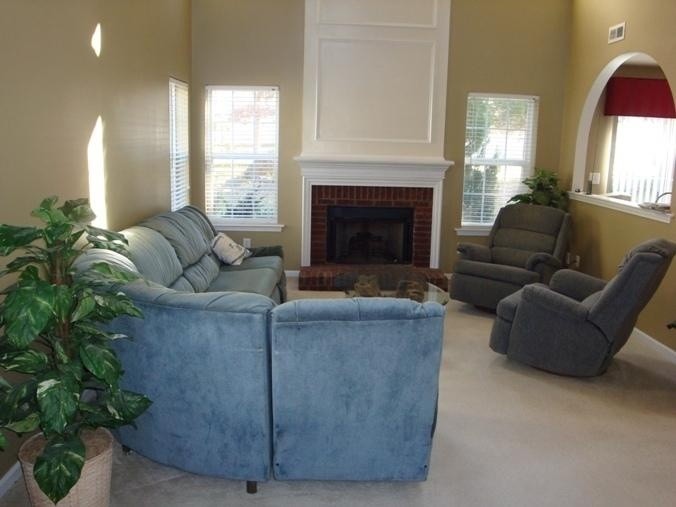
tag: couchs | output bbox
[71,205,285,494]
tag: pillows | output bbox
[209,231,253,270]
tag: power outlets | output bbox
[576,255,580,266]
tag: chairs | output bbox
[447,198,572,319]
[269,292,445,485]
[488,238,675,381]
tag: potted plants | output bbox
[0,195,152,506]
